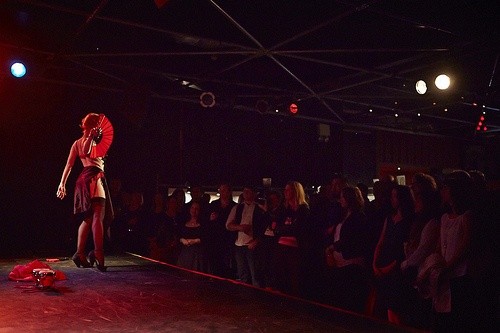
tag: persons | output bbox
[56,112,113,274]
[109,169,500,333]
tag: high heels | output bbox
[88,250,106,272]
[72,253,93,268]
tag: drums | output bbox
[32,268,57,288]
